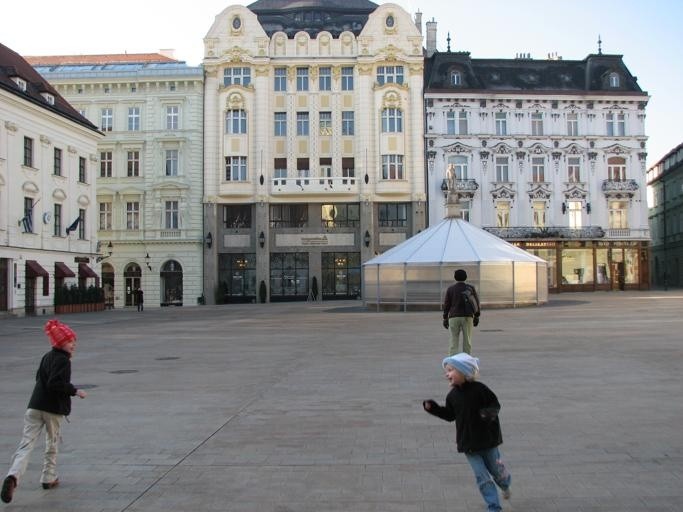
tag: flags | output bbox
[67,216,81,235]
[23,207,32,233]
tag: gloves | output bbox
[473,316,480,327]
[443,318,449,329]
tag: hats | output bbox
[42,318,77,349]
[454,268,467,281]
[442,352,480,380]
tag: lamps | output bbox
[365,230,370,247]
[96,241,112,263]
[259,231,264,248]
[206,232,212,249]
[145,253,151,271]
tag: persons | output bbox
[422,352,511,512]
[134,287,143,312]
[1,320,87,503]
[445,163,457,194]
[443,270,480,357]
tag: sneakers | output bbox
[41,478,59,489]
[0,476,16,503]
[501,485,512,499]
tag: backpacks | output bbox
[461,286,478,316]
